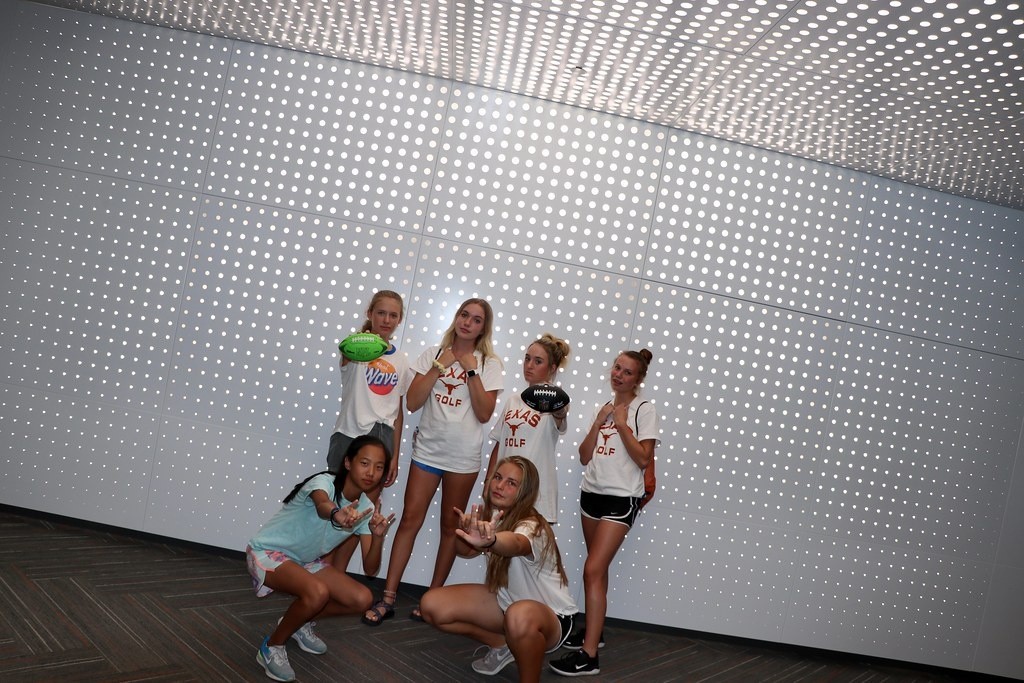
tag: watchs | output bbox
[466,369,479,377]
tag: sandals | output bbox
[361,601,395,627]
[411,605,426,621]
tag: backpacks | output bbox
[635,401,656,508]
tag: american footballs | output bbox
[520,384,570,413]
[338,333,389,362]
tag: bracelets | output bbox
[331,507,342,528]
[482,535,497,548]
[433,360,446,374]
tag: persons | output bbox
[482,334,573,523]
[327,290,408,573]
[361,299,503,625]
[246,435,396,683]
[420,455,579,683]
[548,348,660,676]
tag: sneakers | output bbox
[278,616,327,654]
[548,648,600,677]
[256,637,296,682]
[471,643,515,675]
[562,628,605,649]
